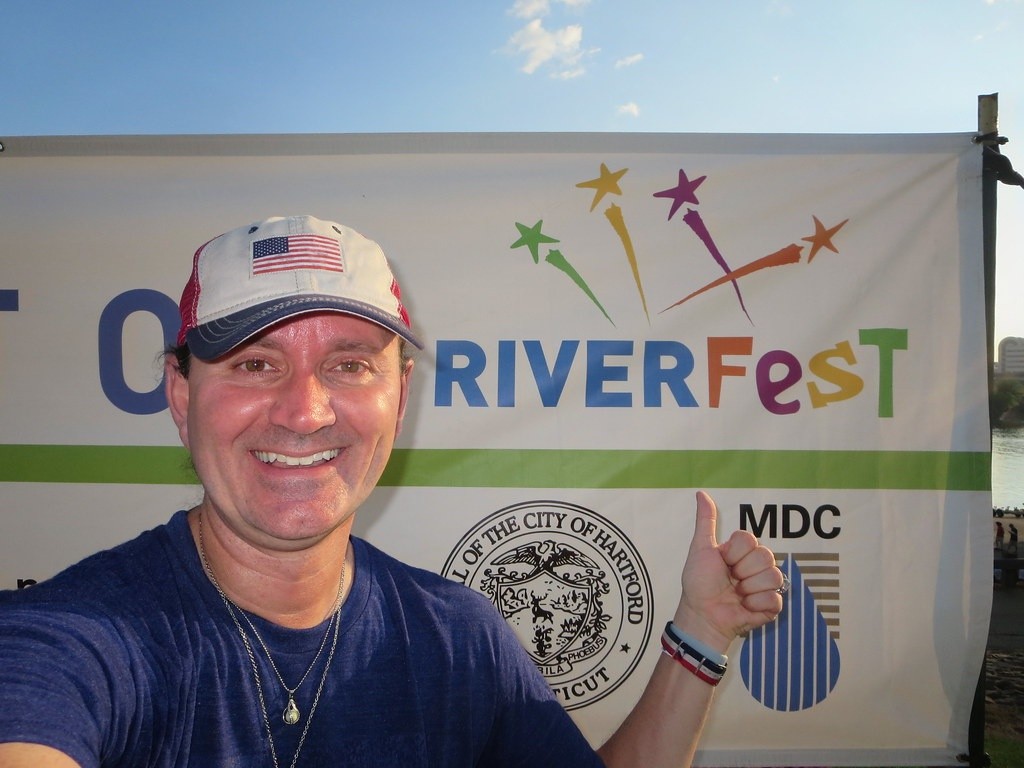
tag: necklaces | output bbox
[198,515,346,768]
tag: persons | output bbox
[0,215,784,768]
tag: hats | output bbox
[178,215,425,363]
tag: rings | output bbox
[777,573,789,595]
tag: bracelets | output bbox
[662,622,729,686]
